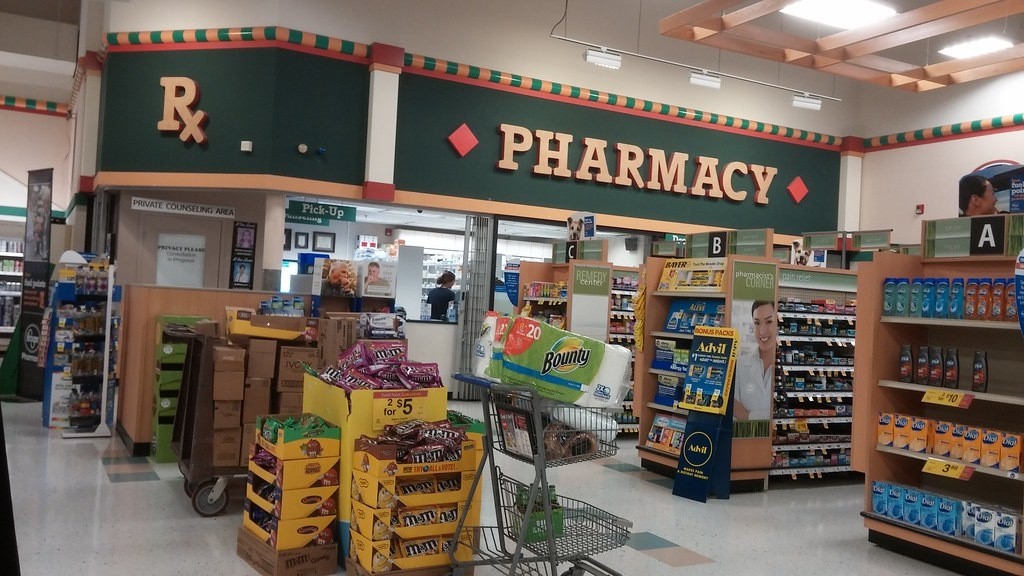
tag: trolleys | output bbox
[446,374,634,576]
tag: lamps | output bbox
[580,47,622,69]
[688,70,721,89]
[790,93,822,111]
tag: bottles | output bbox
[85,266,96,295]
[75,266,84,294]
[516,482,559,513]
[69,388,99,415]
[535,311,544,322]
[71,345,102,376]
[73,304,103,336]
[97,266,108,294]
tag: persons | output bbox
[425,271,457,320]
[732,300,777,420]
[234,264,249,283]
[320,262,355,298]
[362,262,392,297]
[959,175,998,216]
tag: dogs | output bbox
[792,242,812,266]
[568,217,584,240]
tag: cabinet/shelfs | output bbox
[0,214,23,354]
[776,265,859,480]
[643,230,772,493]
[860,250,1024,576]
[61,265,115,439]
[517,261,610,343]
[609,266,642,435]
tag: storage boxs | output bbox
[644,256,727,456]
[872,412,1024,556]
[192,304,487,576]
